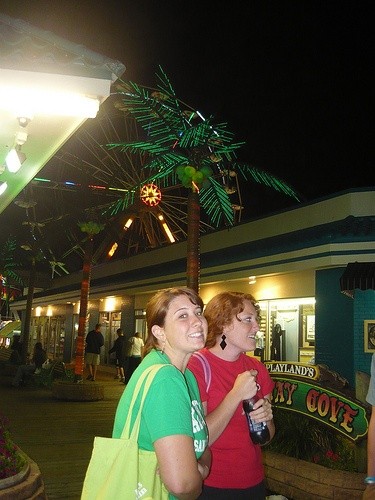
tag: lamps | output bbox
[6,149,26,173]
[0,181,7,195]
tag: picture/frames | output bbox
[364,320,375,353]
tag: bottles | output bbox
[243,394,270,446]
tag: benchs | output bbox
[0,348,73,385]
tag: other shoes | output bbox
[92,378,96,383]
[119,377,125,382]
[89,374,93,380]
[112,375,119,379]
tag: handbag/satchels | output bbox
[81,364,174,499]
